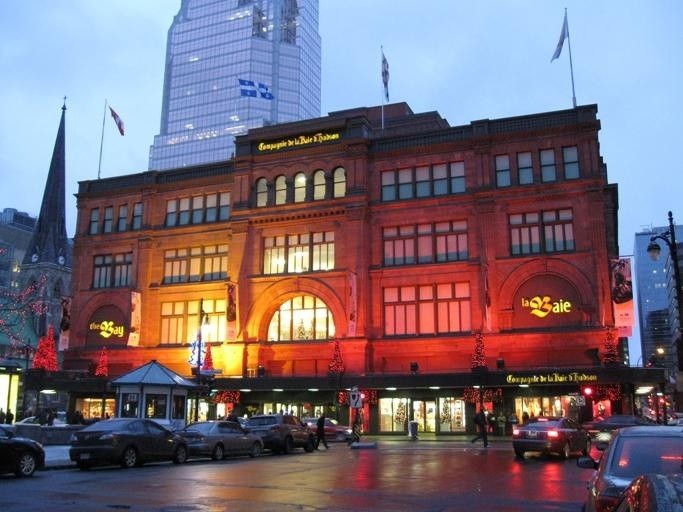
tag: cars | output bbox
[583,426,682,511]
[171,414,354,460]
[0,411,66,476]
[590,415,661,430]
[68,417,189,470]
[512,416,586,459]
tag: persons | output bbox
[6,409,14,424]
[314,413,332,450]
[0,408,5,424]
[522,412,529,425]
[471,406,491,448]
[225,410,241,425]
[497,411,507,437]
[508,411,519,428]
[487,409,496,433]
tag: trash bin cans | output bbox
[408,422,418,438]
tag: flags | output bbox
[238,79,276,100]
[107,107,125,136]
[550,11,568,62]
[381,50,393,103]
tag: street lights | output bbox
[646,210,682,371]
